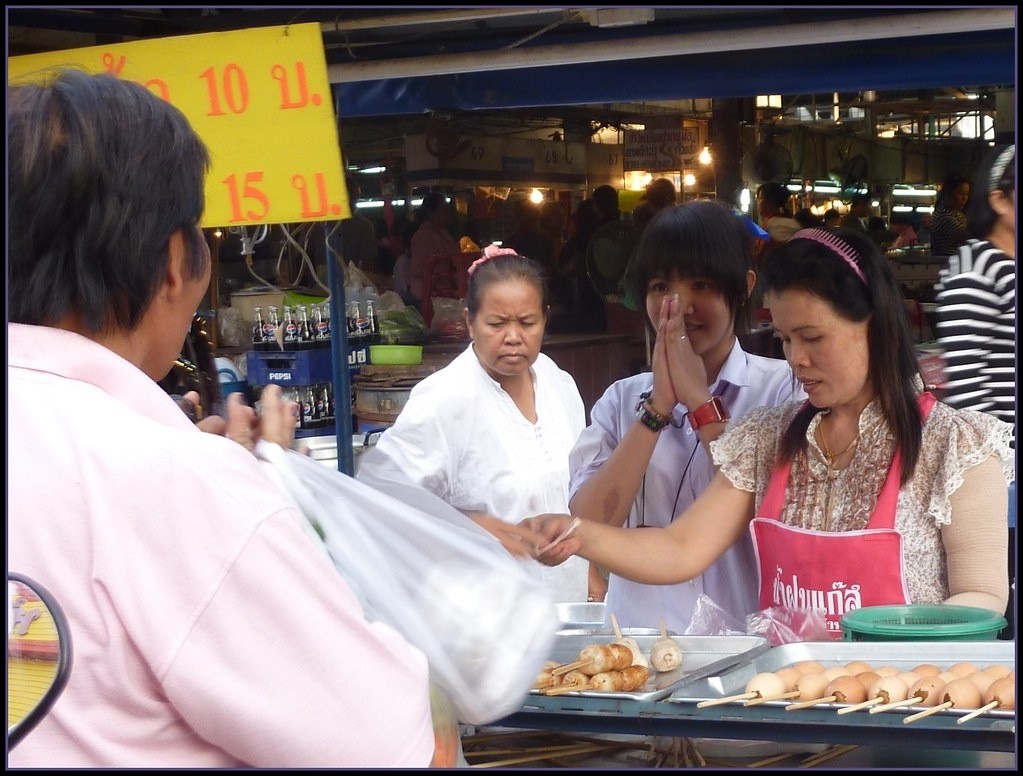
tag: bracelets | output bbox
[640,392,674,420]
[633,403,665,432]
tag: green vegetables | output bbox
[377,312,431,342]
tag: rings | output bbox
[681,335,686,339]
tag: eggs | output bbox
[746,660,1015,710]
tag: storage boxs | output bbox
[246,341,373,387]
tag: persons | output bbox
[516,220,1016,643]
[295,176,415,289]
[418,180,675,330]
[928,170,971,255]
[753,182,918,252]
[567,199,809,635]
[365,240,609,607]
[8,67,458,768]
[934,141,1018,641]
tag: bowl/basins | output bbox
[369,344,424,365]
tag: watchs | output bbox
[687,396,731,430]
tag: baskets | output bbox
[839,605,1007,643]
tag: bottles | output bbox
[250,299,378,429]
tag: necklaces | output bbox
[816,423,861,459]
